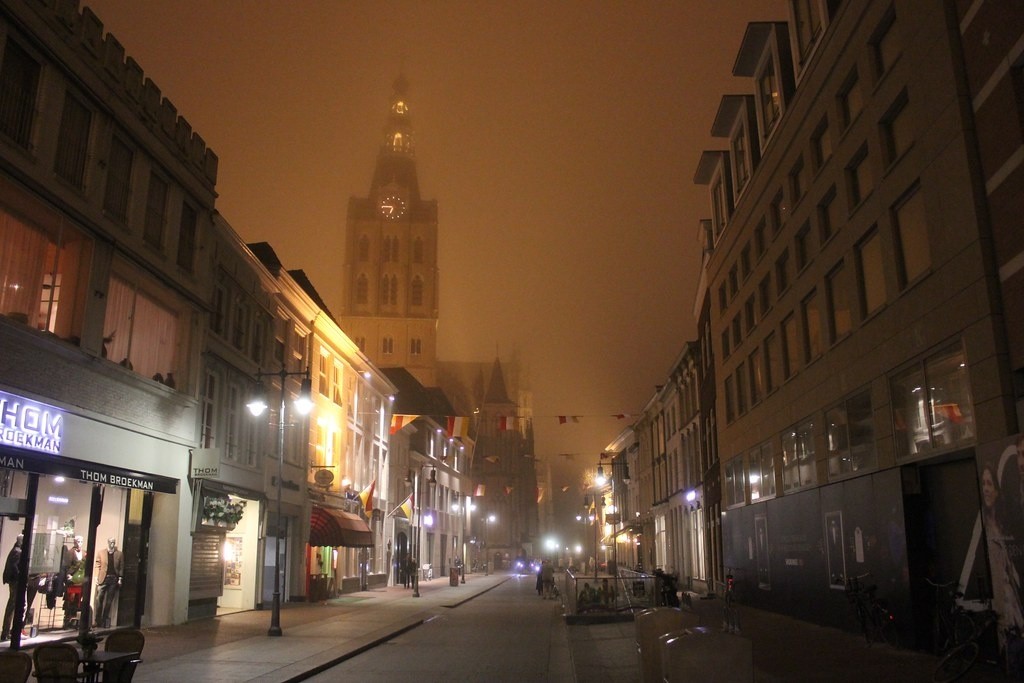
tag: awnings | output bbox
[309,505,376,549]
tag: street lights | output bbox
[594,454,632,580]
[452,492,479,587]
[241,362,315,639]
[402,462,438,597]
[584,492,607,578]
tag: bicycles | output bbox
[720,565,745,635]
[833,575,900,649]
[924,576,998,683]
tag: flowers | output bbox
[202,495,248,526]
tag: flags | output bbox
[558,415,631,424]
[481,455,502,468]
[497,417,515,431]
[933,403,964,425]
[503,487,543,503]
[389,415,416,436]
[893,410,914,433]
[401,494,414,526]
[447,416,470,438]
[473,484,485,497]
[359,481,376,518]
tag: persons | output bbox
[980,432,1024,683]
[62,536,87,630]
[0,533,28,641]
[651,566,681,608]
[92,537,124,628]
[454,555,461,575]
[579,579,616,608]
[402,553,416,589]
[535,561,555,599]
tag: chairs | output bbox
[0,629,145,683]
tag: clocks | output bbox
[381,196,406,220]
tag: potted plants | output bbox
[76,628,103,653]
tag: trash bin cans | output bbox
[309,573,328,602]
[450,568,458,586]
[488,562,494,574]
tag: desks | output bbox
[28,650,140,683]
